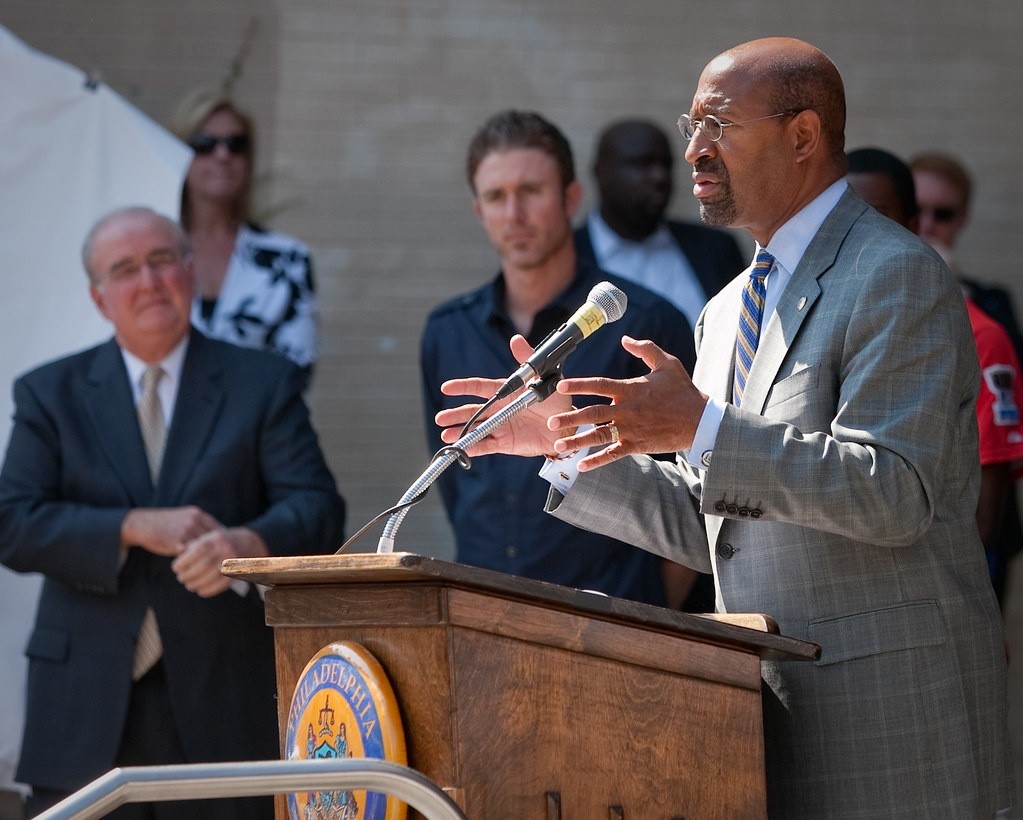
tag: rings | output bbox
[606,423,619,443]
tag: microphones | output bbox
[495,280,628,400]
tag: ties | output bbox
[733,250,774,415]
[133,368,169,681]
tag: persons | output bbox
[414,109,700,605]
[562,118,749,330]
[831,147,1023,555]
[158,96,329,388]
[0,206,334,820]
[909,155,1023,366]
[442,35,1023,820]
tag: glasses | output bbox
[677,107,807,141]
[918,205,957,221]
[189,131,251,154]
